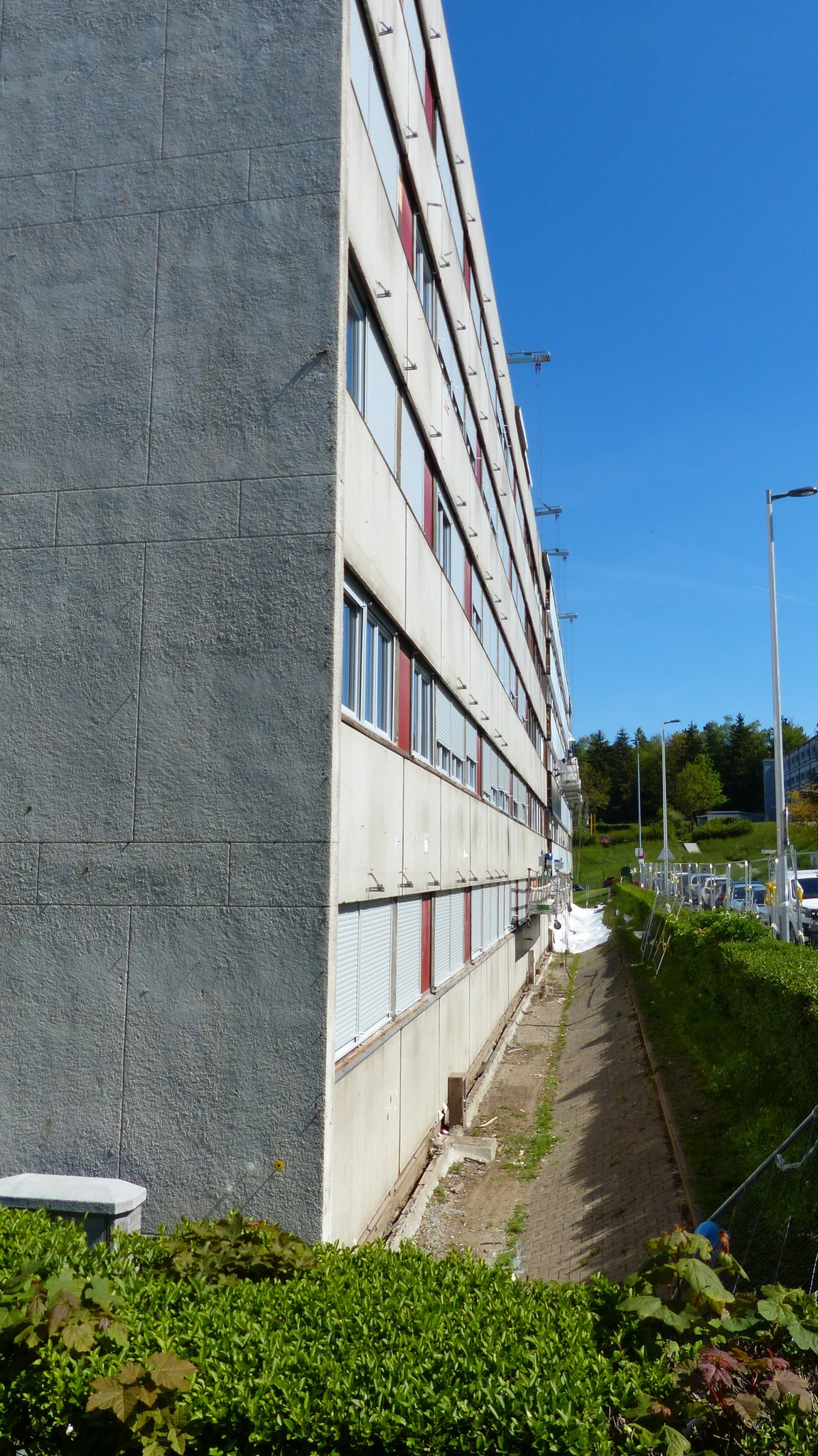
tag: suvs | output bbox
[766,869,818,947]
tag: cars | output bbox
[651,871,767,924]
[603,876,640,888]
[573,883,584,892]
[598,835,610,843]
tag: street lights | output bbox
[661,718,681,896]
[636,740,651,888]
[765,485,818,944]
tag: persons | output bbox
[702,868,707,873]
[690,867,696,873]
